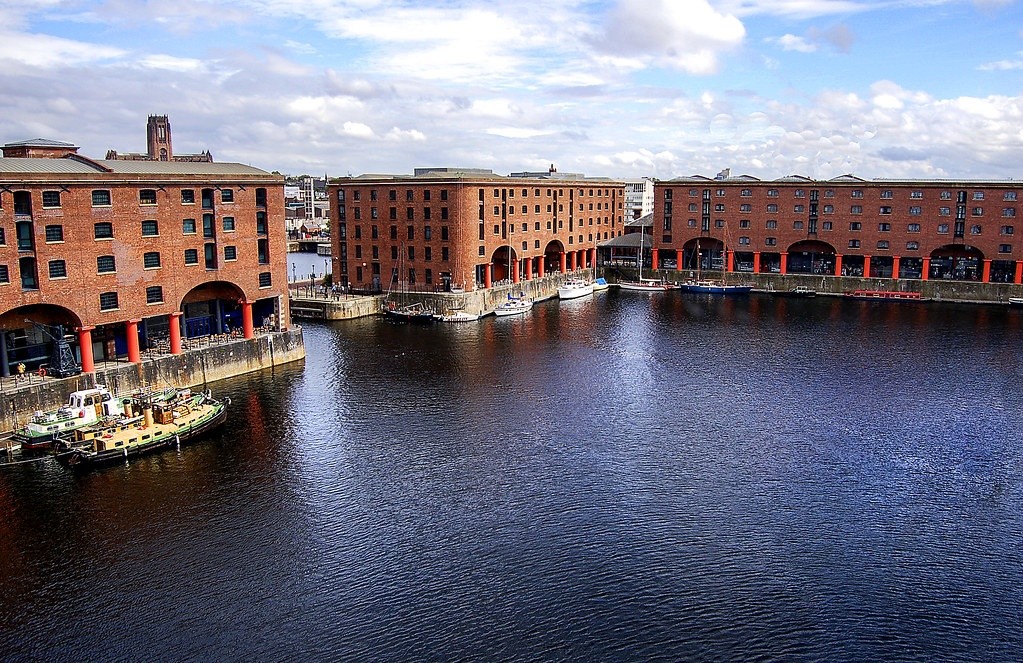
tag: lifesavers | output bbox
[38,369,47,377]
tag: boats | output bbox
[23,388,139,450]
[1007,297,1022,306]
[61,389,231,468]
[792,286,816,296]
[433,271,478,324]
[593,277,609,291]
[557,271,593,299]
[844,288,934,303]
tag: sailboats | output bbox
[493,228,537,317]
[681,221,755,295]
[616,223,681,292]
[379,242,437,324]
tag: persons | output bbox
[331,283,336,291]
[766,281,773,290]
[340,285,344,294]
[349,283,353,292]
[20,361,26,371]
[319,283,323,292]
[435,283,439,293]
[324,284,328,293]
[17,362,24,377]
